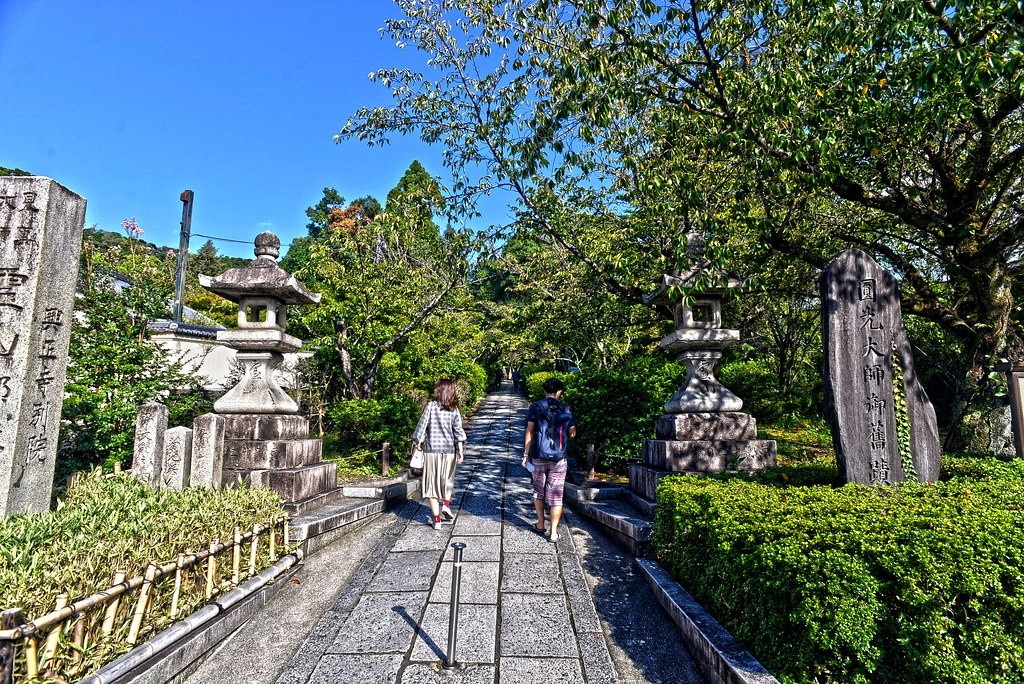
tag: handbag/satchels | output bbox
[409,445,423,469]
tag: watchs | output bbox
[522,452,528,457]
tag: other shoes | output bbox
[442,505,454,520]
[432,521,441,530]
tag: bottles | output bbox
[525,460,535,472]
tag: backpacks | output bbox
[535,399,573,460]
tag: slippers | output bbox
[549,532,561,542]
[531,523,546,532]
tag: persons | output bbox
[410,379,468,530]
[493,368,503,391]
[512,368,520,391]
[520,377,576,543]
